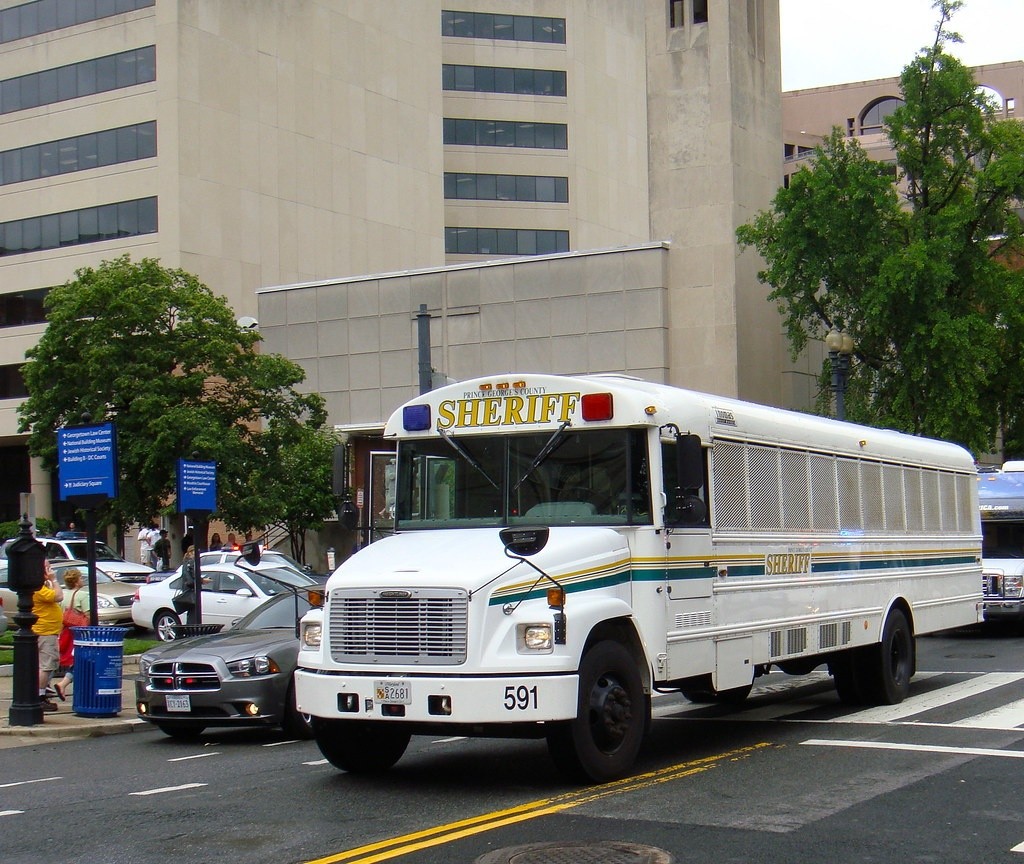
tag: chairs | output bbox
[468,462,515,517]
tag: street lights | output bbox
[829,319,855,419]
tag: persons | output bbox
[210,532,253,553]
[32,559,91,711]
[181,528,193,554]
[138,520,172,571]
[182,544,214,625]
[68,522,77,532]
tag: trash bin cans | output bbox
[170,624,225,640]
[69,625,130,717]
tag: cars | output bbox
[0,563,138,633]
[132,560,320,642]
[1,532,155,584]
[145,545,309,583]
[134,583,328,741]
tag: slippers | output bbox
[53,684,66,702]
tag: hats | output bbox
[160,530,168,535]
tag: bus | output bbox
[977,461,1024,617]
[291,370,982,784]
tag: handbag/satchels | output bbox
[59,607,90,667]
[172,587,197,615]
[156,557,164,572]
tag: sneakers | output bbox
[38,698,58,713]
[44,683,61,699]
